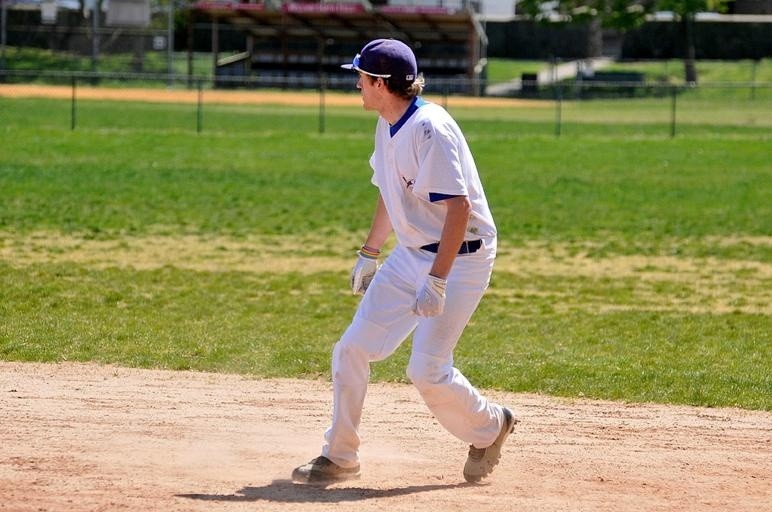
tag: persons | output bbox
[287,35,521,488]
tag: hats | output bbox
[340,38,417,83]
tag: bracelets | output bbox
[359,245,381,261]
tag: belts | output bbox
[410,275,447,318]
[422,240,482,254]
[350,251,378,297]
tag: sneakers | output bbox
[464,406,520,481]
[292,457,360,483]
